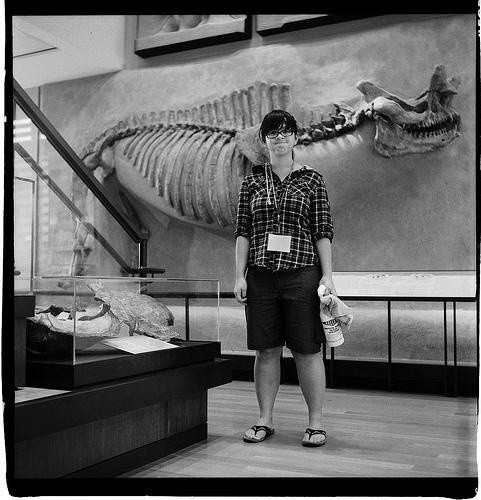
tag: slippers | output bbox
[243,420,275,444]
[301,424,329,448]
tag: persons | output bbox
[233,109,338,448]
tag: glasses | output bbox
[264,129,295,139]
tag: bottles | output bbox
[316,285,345,348]
